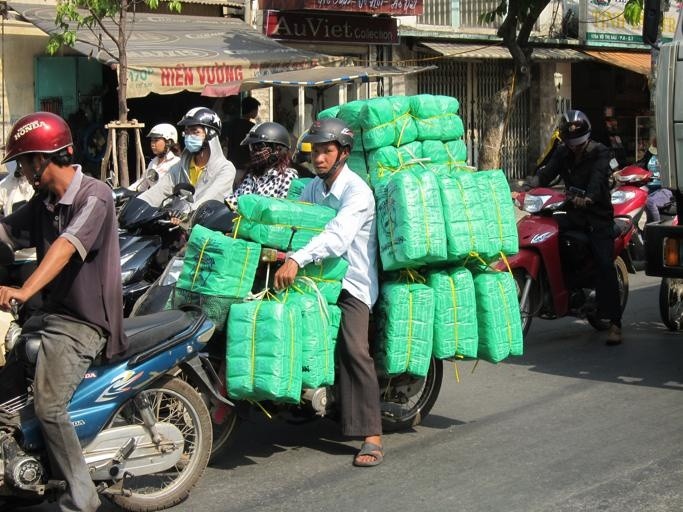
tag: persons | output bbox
[225,97,261,190]
[272,117,385,467]
[610,135,675,225]
[511,110,624,346]
[0,111,130,512]
[225,121,299,226]
[125,122,180,193]
[138,106,237,267]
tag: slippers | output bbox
[353,443,384,467]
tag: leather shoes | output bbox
[606,324,621,344]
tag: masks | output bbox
[250,147,272,167]
[183,134,205,153]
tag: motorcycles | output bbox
[609,157,683,330]
[489,182,629,340]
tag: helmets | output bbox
[146,123,177,143]
[558,110,591,146]
[301,117,353,151]
[176,106,222,137]
[1,111,74,164]
[239,121,291,149]
